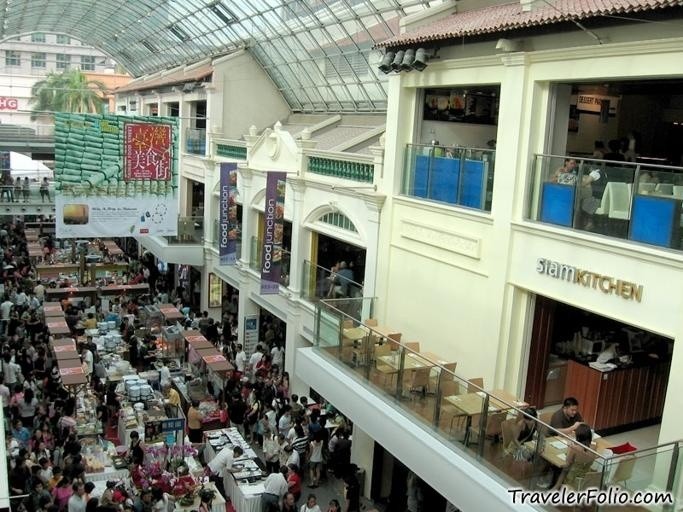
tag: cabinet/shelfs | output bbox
[566,351,670,434]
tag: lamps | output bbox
[377,43,443,74]
[328,183,377,210]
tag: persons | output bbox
[595,342,628,364]
[508,408,543,462]
[619,139,635,161]
[39,176,52,203]
[0,213,422,511]
[579,159,607,231]
[552,157,576,181]
[541,398,584,481]
[591,139,607,159]
[537,425,595,494]
[0,174,30,203]
[601,137,624,168]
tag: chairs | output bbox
[451,146,683,234]
